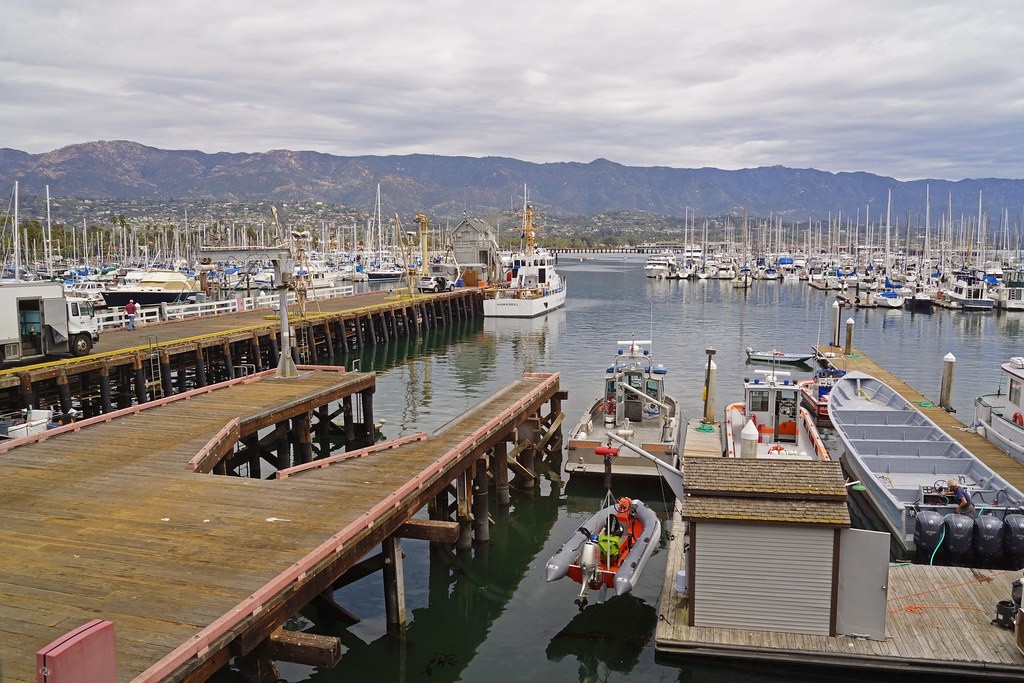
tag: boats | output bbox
[542,499,662,606]
[972,357,1024,471]
[797,369,848,427]
[466,181,571,318]
[744,345,814,366]
[101,270,205,305]
[564,339,688,489]
[724,353,831,458]
[0,405,78,448]
[823,369,1024,564]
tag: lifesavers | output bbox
[767,446,785,455]
[1011,413,1023,426]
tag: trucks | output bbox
[0,283,100,368]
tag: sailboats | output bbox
[3,181,452,281]
[644,180,1024,325]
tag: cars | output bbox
[415,276,457,293]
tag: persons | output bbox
[940,478,975,515]
[123,300,138,331]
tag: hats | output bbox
[129,300,134,303]
